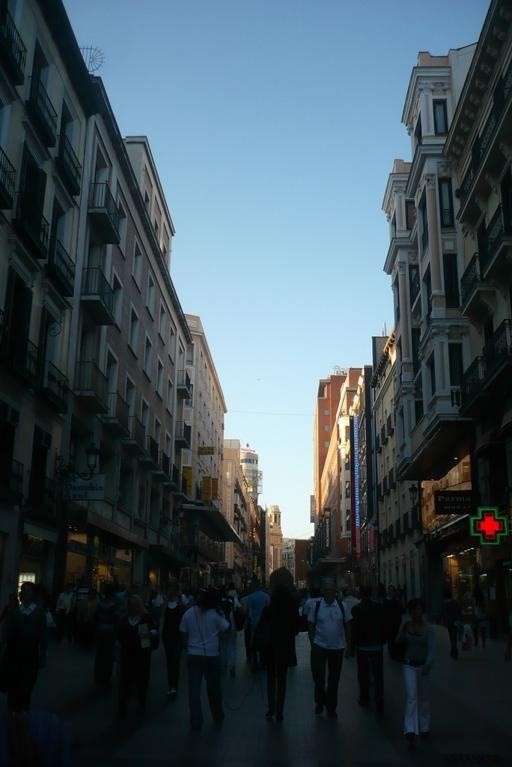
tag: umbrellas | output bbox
[327,709,338,718]
[315,705,324,713]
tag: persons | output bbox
[469,587,487,649]
[306,583,350,718]
[150,578,407,693]
[253,578,301,722]
[395,599,434,742]
[178,589,232,734]
[2,579,103,715]
[351,584,390,729]
[112,595,159,722]
[444,598,462,651]
[94,585,124,689]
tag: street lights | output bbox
[95,623,114,638]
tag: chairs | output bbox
[166,688,176,700]
[407,734,417,746]
[421,731,434,738]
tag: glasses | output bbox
[64,441,101,480]
[164,504,185,524]
